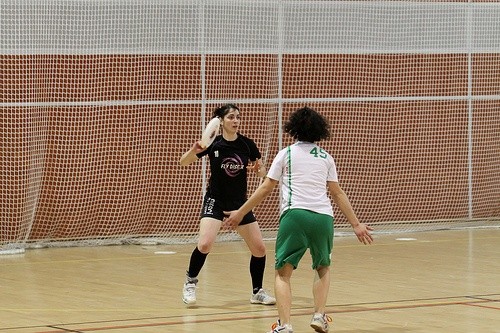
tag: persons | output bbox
[222,107,374,333]
[180,104,275,305]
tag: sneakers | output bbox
[271,320,292,333]
[182,279,198,306]
[250,291,276,304]
[310,313,331,333]
